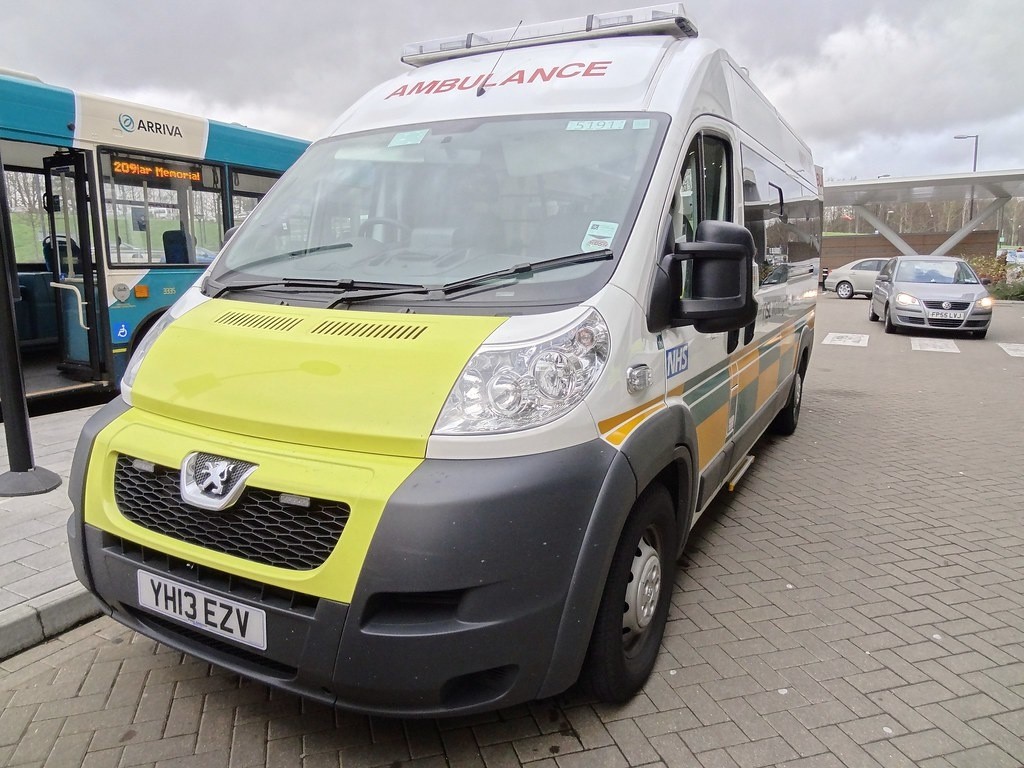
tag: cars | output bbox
[824,258,891,299]
[160,246,217,264]
[91,243,148,258]
[234,211,251,218]
[868,255,993,340]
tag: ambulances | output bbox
[66,2,826,722]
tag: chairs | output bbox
[162,230,192,264]
[42,235,83,274]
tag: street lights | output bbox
[953,134,979,222]
[876,175,890,217]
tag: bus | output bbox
[0,70,312,422]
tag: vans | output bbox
[150,208,171,220]
